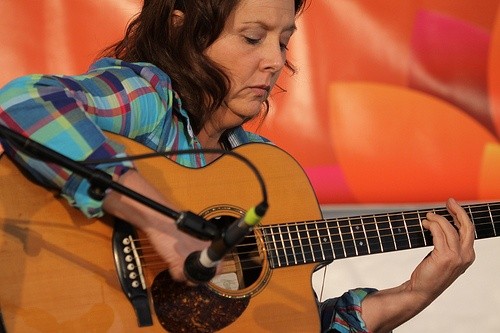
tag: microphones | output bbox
[183,200,269,284]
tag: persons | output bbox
[1,1,476,332]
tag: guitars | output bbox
[1,142,499,333]
